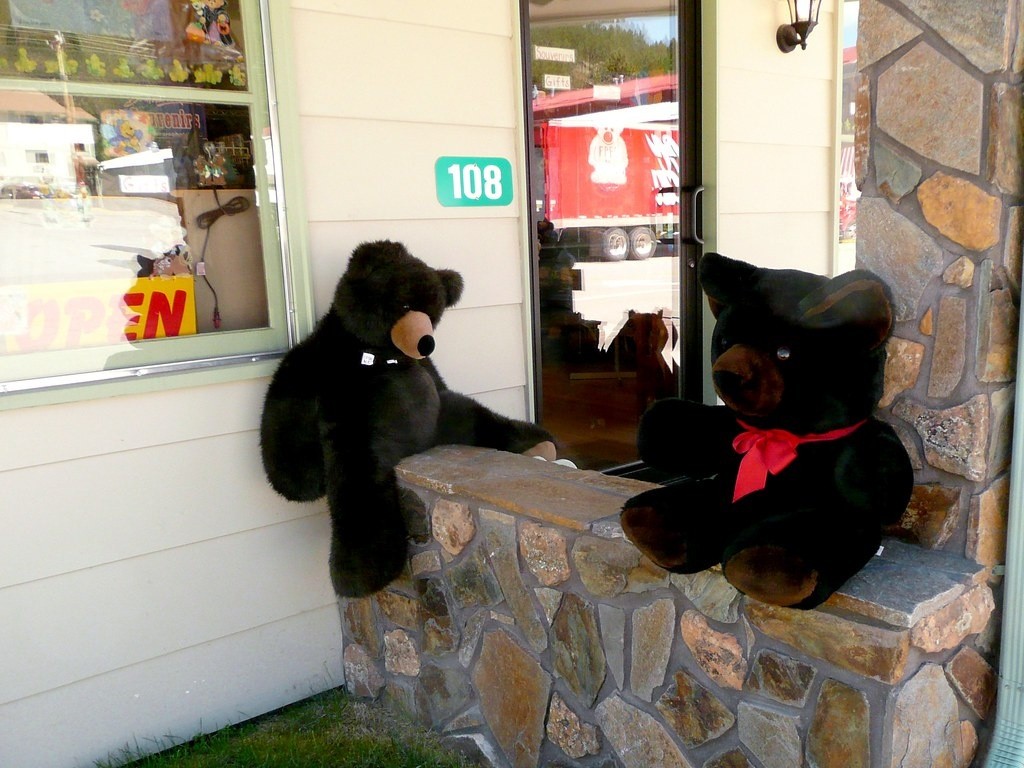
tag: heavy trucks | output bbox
[536,115,857,259]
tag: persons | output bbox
[534,218,578,315]
[72,143,101,224]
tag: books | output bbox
[8,1,177,42]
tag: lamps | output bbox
[777,0,822,54]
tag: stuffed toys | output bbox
[617,249,914,609]
[257,236,560,600]
[535,311,673,400]
